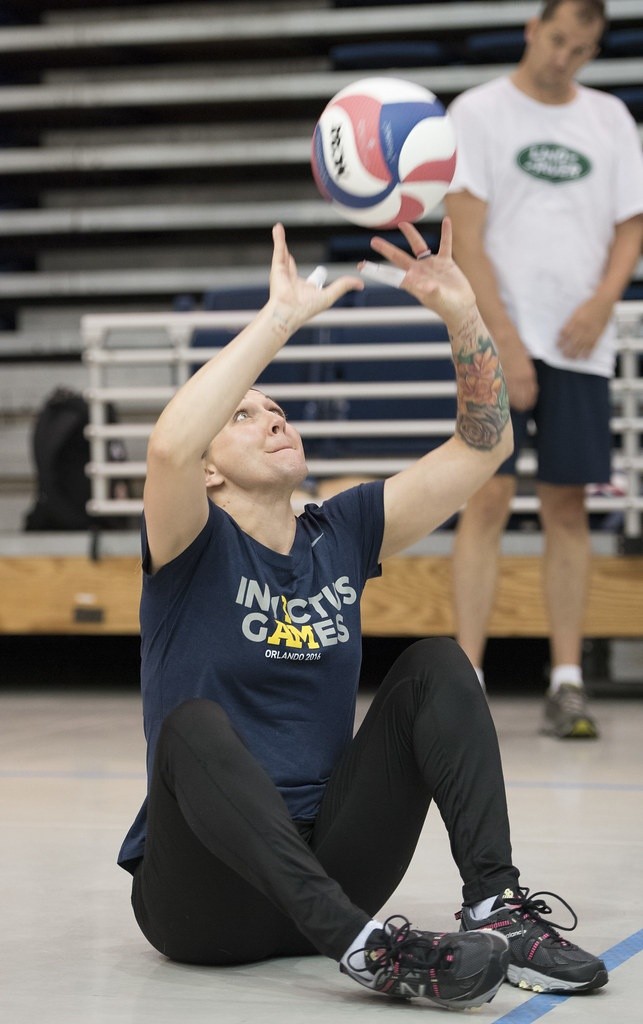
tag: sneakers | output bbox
[536,676,601,745]
[338,914,513,1013]
[455,888,609,997]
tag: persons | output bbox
[446,1,643,737]
[116,215,608,1009]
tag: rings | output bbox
[418,250,431,260]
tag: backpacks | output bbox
[19,383,134,537]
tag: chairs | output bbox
[173,285,460,514]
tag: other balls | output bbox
[308,73,462,229]
